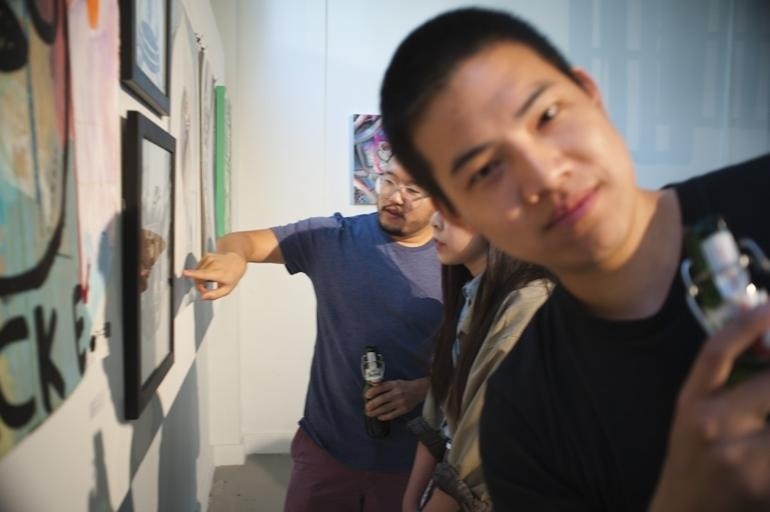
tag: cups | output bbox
[360,352,386,387]
[676,238,770,363]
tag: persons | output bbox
[395,205,558,509]
[379,4,770,509]
[175,150,469,509]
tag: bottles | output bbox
[687,217,770,387]
[361,344,395,441]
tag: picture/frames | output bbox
[122,1,176,420]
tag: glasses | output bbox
[374,173,431,204]
